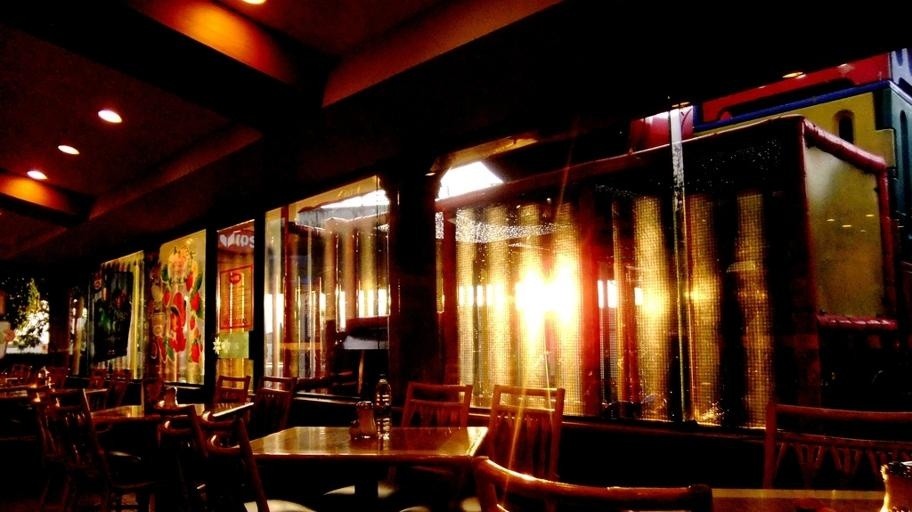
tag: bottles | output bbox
[349,400,375,439]
[374,378,392,440]
